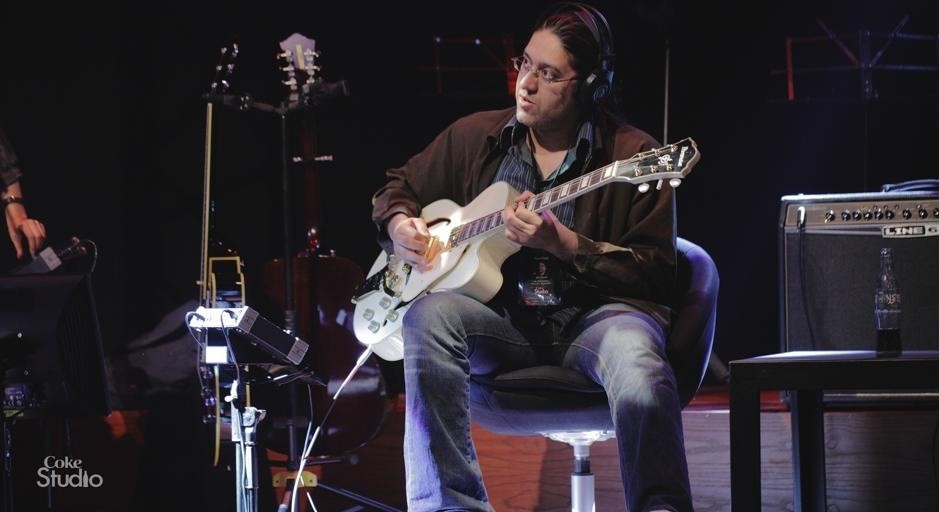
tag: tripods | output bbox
[277,100,405,512]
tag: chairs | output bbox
[467,237,720,512]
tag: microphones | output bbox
[281,80,356,114]
[205,92,255,111]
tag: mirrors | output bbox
[728,350,939,512]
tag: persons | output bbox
[372,2,694,512]
[536,261,547,275]
[0,127,47,260]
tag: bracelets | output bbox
[1,195,24,211]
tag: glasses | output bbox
[510,56,576,82]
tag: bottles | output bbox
[874,247,902,355]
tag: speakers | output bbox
[776,188,937,409]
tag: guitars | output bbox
[260,34,387,460]
[199,43,253,468]
[352,137,701,361]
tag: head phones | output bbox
[560,1,617,115]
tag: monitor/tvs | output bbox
[0,273,114,512]
